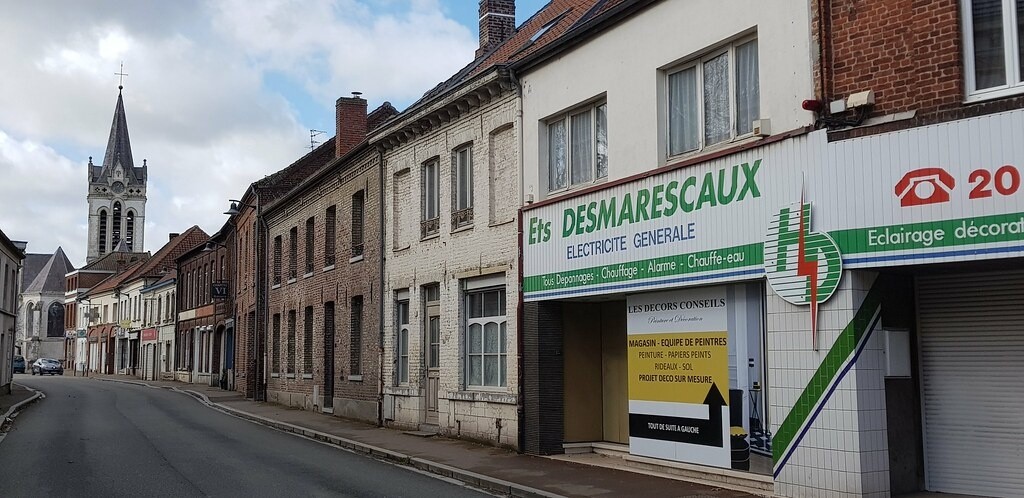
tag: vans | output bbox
[13,355,26,374]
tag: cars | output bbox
[32,357,65,376]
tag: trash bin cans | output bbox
[76,363,83,376]
